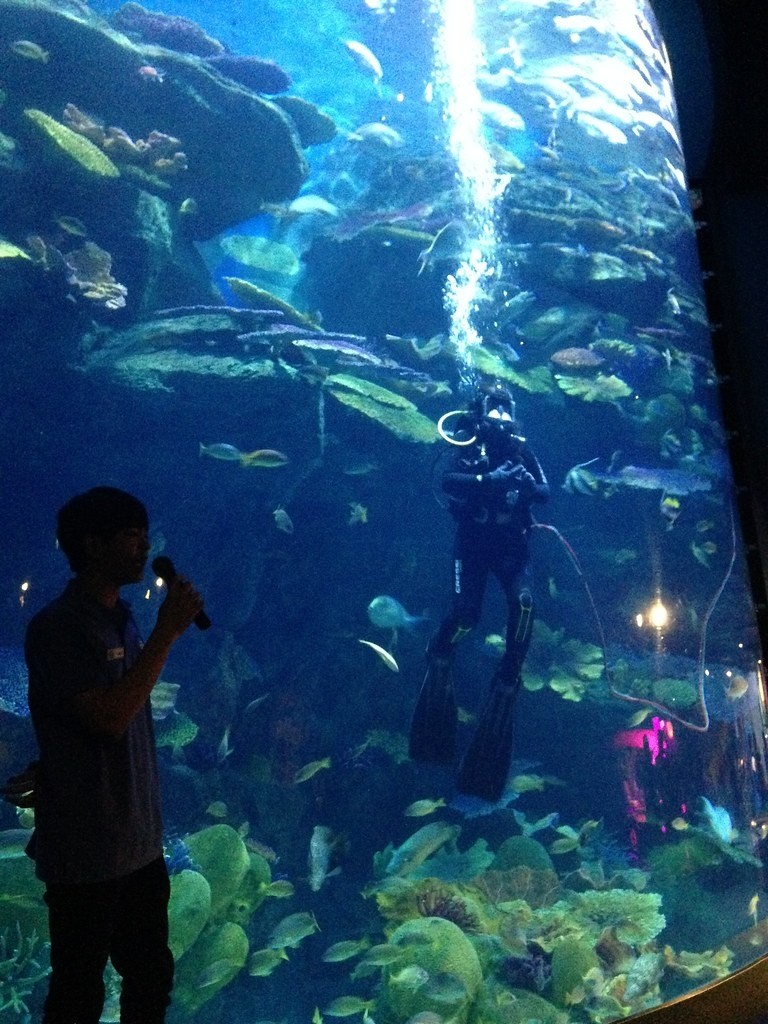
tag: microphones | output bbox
[152,556,211,631]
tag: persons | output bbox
[21,485,212,1024]
[401,382,553,804]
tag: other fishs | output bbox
[387,966,429,995]
[139,66,167,83]
[273,508,294,534]
[197,443,289,468]
[416,220,471,278]
[265,880,296,897]
[320,938,369,962]
[268,909,322,949]
[350,943,407,980]
[12,40,49,65]
[701,795,733,845]
[248,947,290,978]
[725,675,749,699]
[217,728,235,763]
[366,595,418,632]
[581,944,736,1019]
[306,825,343,892]
[385,820,462,879]
[50,209,88,238]
[358,639,399,673]
[205,801,229,818]
[293,756,332,785]
[341,458,381,474]
[347,123,400,147]
[344,40,383,85]
[534,123,561,165]
[194,958,240,990]
[403,796,448,818]
[311,996,376,1024]
[690,520,719,570]
